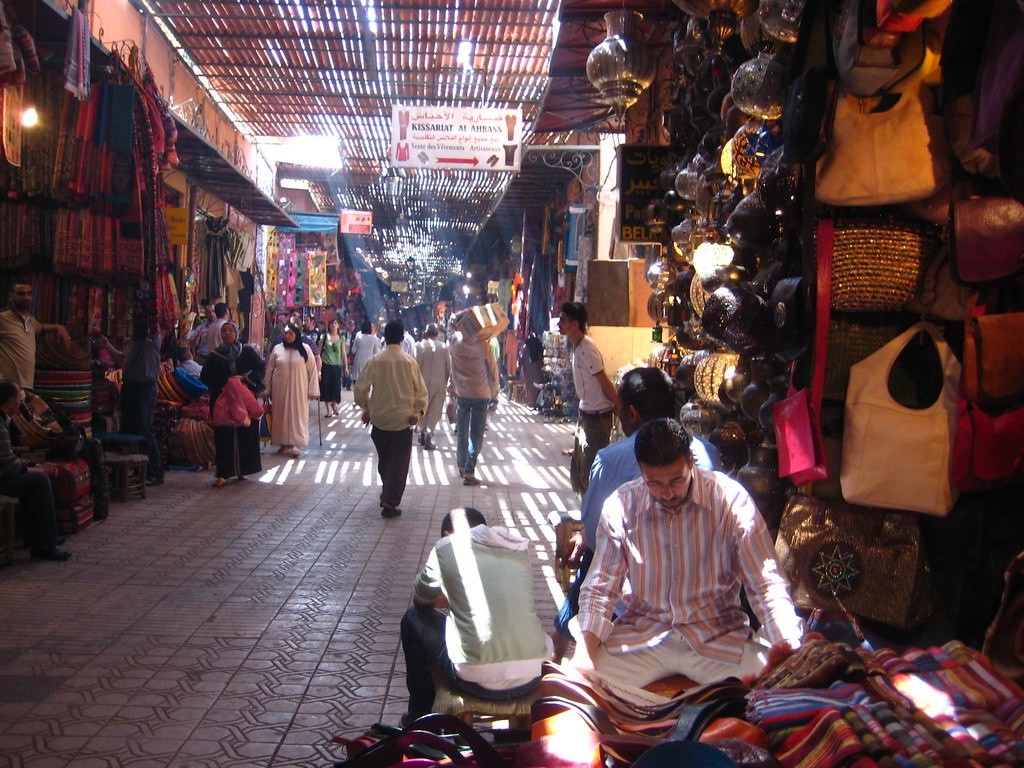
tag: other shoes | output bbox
[278,445,290,454]
[463,477,481,485]
[459,468,465,477]
[32,549,71,560]
[292,447,300,456]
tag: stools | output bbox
[0,494,20,568]
[102,452,149,501]
[548,508,582,593]
[450,694,534,744]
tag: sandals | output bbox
[213,477,229,487]
[331,403,338,415]
[325,412,332,418]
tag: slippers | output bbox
[381,507,402,517]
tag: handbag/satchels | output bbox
[447,397,457,424]
[769,0,1023,634]
[212,378,263,427]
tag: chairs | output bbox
[507,366,527,402]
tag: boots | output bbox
[424,436,436,450]
[418,433,425,445]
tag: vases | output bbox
[585,0,830,502]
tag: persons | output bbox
[568,417,807,690]
[0,280,71,390]
[447,308,500,485]
[178,347,203,376]
[264,324,320,456]
[317,319,349,418]
[315,320,327,335]
[396,318,450,450]
[269,317,322,372]
[290,308,300,317]
[191,302,239,365]
[401,508,548,731]
[338,319,385,391]
[580,367,723,623]
[0,380,72,560]
[201,321,266,486]
[354,321,429,517]
[557,301,619,496]
[302,314,320,345]
[102,321,165,486]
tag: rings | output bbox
[69,337,71,339]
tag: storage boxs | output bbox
[457,303,509,343]
